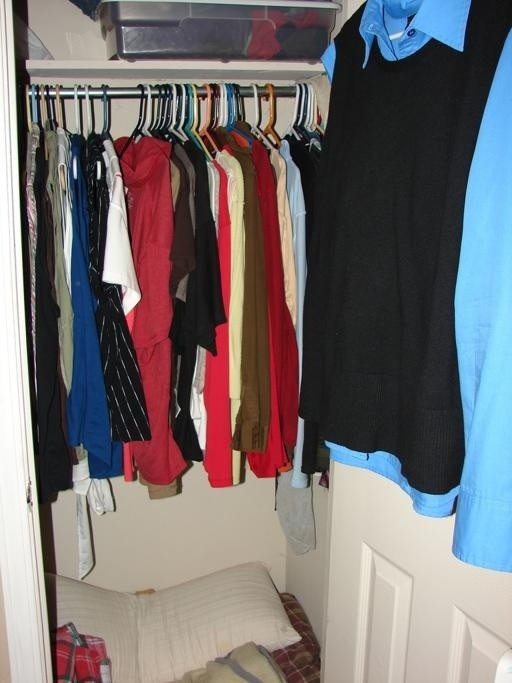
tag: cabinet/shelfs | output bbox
[10,0,335,682]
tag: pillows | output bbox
[46,560,301,682]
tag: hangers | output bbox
[383,2,422,41]
[24,81,329,160]
